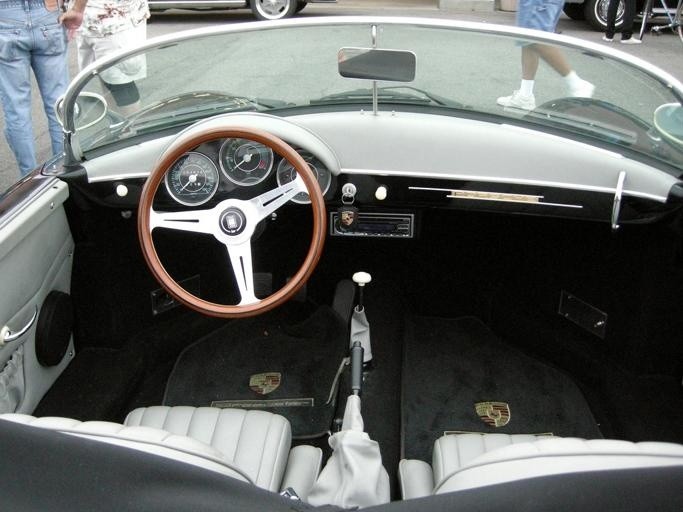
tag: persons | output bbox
[62,0,151,139]
[496,1,595,112]
[603,0,643,45]
[1,0,90,178]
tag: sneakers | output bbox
[565,80,595,97]
[602,36,614,42]
[496,91,535,111]
[620,37,642,44]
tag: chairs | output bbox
[398,434,683,500]
[0,406,323,504]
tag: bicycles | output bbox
[639,1,683,43]
